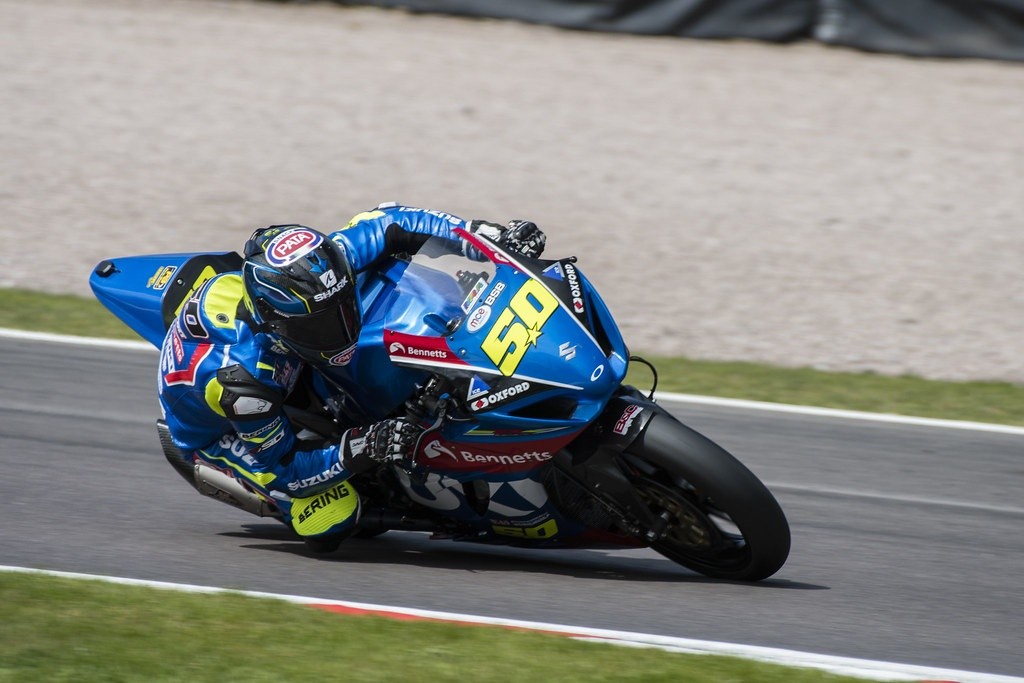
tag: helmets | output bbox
[243,224,360,368]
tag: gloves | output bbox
[337,419,418,473]
[462,219,546,264]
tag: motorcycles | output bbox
[87,221,790,584]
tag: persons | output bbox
[156,201,546,544]
